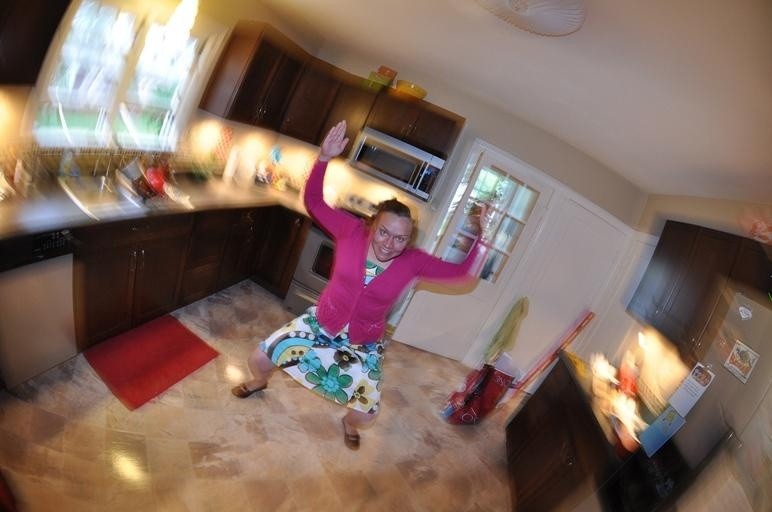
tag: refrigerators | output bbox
[674,292,772,492]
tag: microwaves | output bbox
[349,125,448,204]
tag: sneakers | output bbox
[231,380,268,398]
[342,415,360,450]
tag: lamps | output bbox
[474,1,596,40]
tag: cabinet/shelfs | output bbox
[77,220,185,340]
[367,83,467,160]
[252,204,312,299]
[502,360,623,512]
[0,0,73,89]
[624,220,741,360]
[180,205,273,304]
[276,54,383,159]
[200,17,310,131]
[689,240,770,363]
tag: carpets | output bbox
[81,311,221,411]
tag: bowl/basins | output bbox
[396,79,427,100]
[367,65,397,85]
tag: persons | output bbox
[232,120,491,451]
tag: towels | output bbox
[482,296,530,363]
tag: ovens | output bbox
[284,222,336,318]
